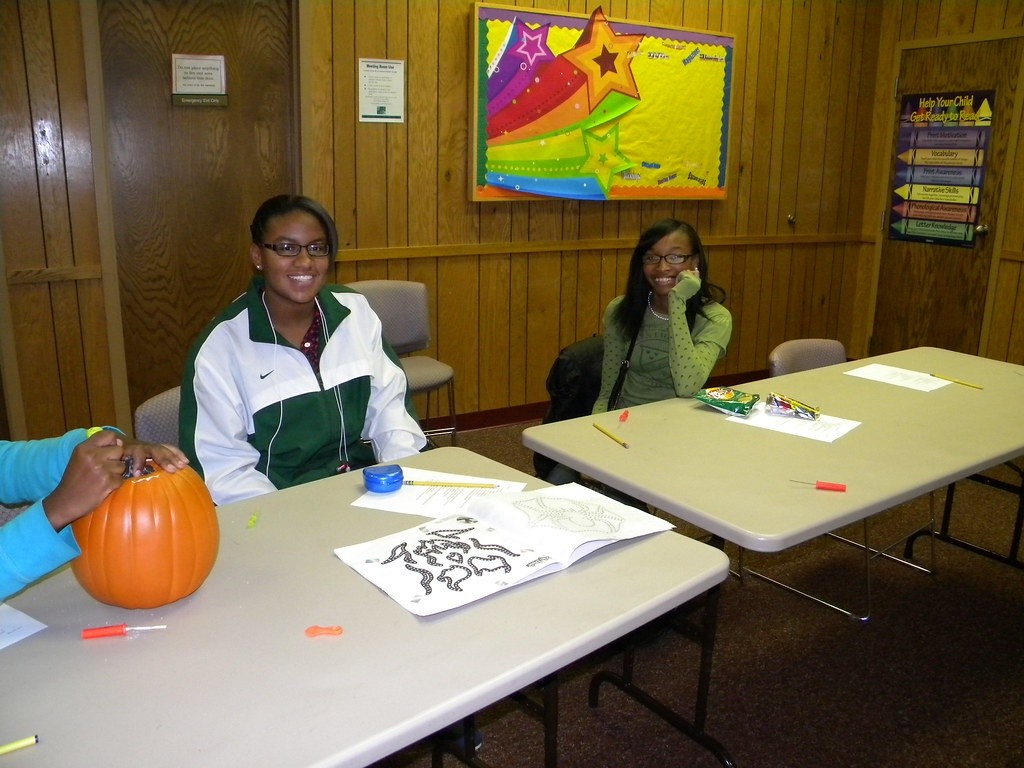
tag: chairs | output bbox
[533,335,603,477]
[133,385,180,448]
[343,279,457,445]
[741,339,937,624]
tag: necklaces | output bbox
[648,291,669,320]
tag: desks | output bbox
[516,346,1024,768]
[0,446,730,768]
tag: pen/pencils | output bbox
[592,421,631,450]
[402,479,502,489]
[930,372,984,390]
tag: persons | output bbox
[177,192,483,755]
[0,426,189,602]
[547,217,732,513]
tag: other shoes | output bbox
[449,722,482,751]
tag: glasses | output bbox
[259,242,330,257]
[641,253,696,264]
